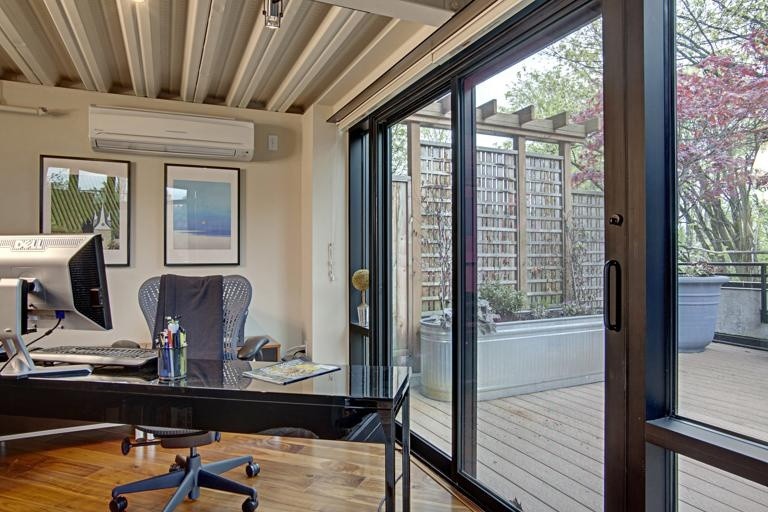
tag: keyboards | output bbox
[27,346,161,367]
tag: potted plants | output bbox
[351,266,373,327]
[415,280,608,405]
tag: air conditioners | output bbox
[88,102,258,164]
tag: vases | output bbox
[677,276,731,355]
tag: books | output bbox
[241,355,344,386]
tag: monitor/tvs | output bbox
[0,233,113,381]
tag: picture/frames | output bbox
[162,161,242,268]
[38,154,133,270]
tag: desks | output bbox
[257,332,285,362]
[2,343,415,511]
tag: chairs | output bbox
[106,271,262,511]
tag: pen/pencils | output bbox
[154,312,187,382]
